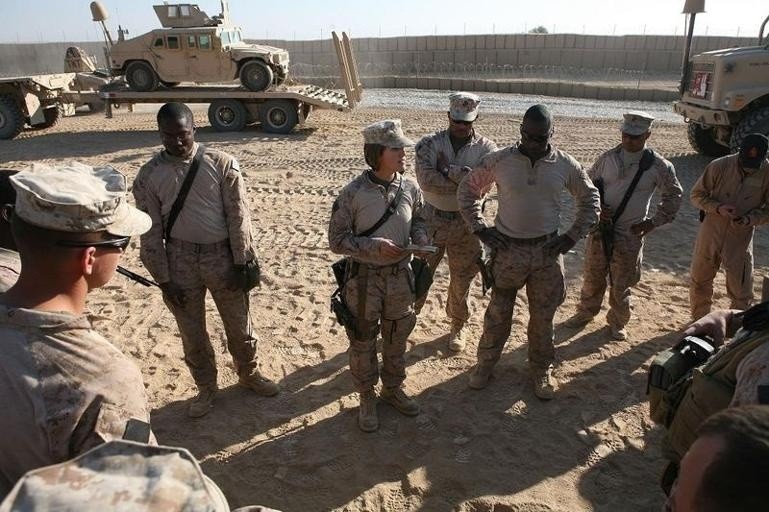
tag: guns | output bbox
[592,175,614,287]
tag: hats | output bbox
[739,133,769,169]
[447,92,482,122]
[0,439,231,512]
[360,118,417,149]
[7,161,153,237]
[620,114,654,136]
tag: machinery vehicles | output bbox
[82,0,291,92]
[670,1,767,167]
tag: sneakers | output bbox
[238,367,278,396]
[449,329,466,350]
[564,312,589,328]
[380,387,419,416]
[469,365,491,389]
[358,392,378,432]
[610,325,628,340]
[529,367,554,400]
[187,384,218,418]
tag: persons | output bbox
[564,110,683,340]
[457,104,601,400]
[328,118,432,432]
[645,277,769,512]
[690,133,769,321]
[413,92,499,352]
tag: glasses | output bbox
[521,126,553,143]
[56,236,131,255]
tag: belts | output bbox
[510,229,558,245]
[423,202,462,221]
